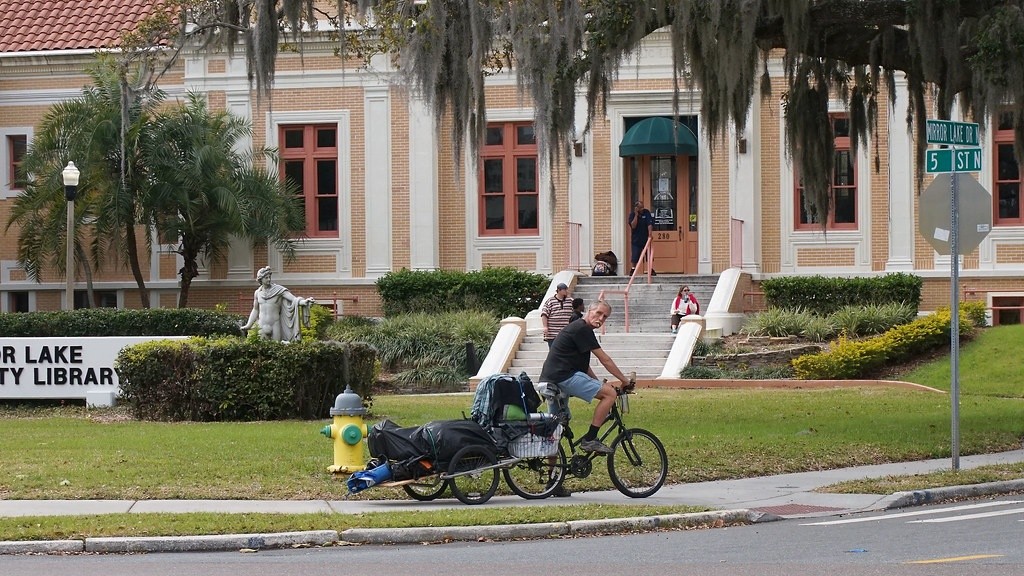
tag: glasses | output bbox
[684,289,690,293]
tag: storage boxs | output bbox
[508,425,563,457]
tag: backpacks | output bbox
[594,261,609,275]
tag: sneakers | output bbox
[547,480,571,497]
[580,438,614,453]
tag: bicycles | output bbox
[500,379,669,499]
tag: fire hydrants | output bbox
[320,385,380,480]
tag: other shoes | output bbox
[651,269,655,276]
[672,329,676,333]
[630,268,635,277]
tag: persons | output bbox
[628,201,657,276]
[539,300,631,497]
[540,283,574,349]
[240,266,316,343]
[670,285,699,332]
[569,298,584,324]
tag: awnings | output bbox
[619,117,698,158]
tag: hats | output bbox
[558,283,567,291]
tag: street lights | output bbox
[61,160,81,312]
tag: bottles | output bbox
[684,295,688,302]
[528,413,555,420]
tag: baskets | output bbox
[508,426,561,458]
